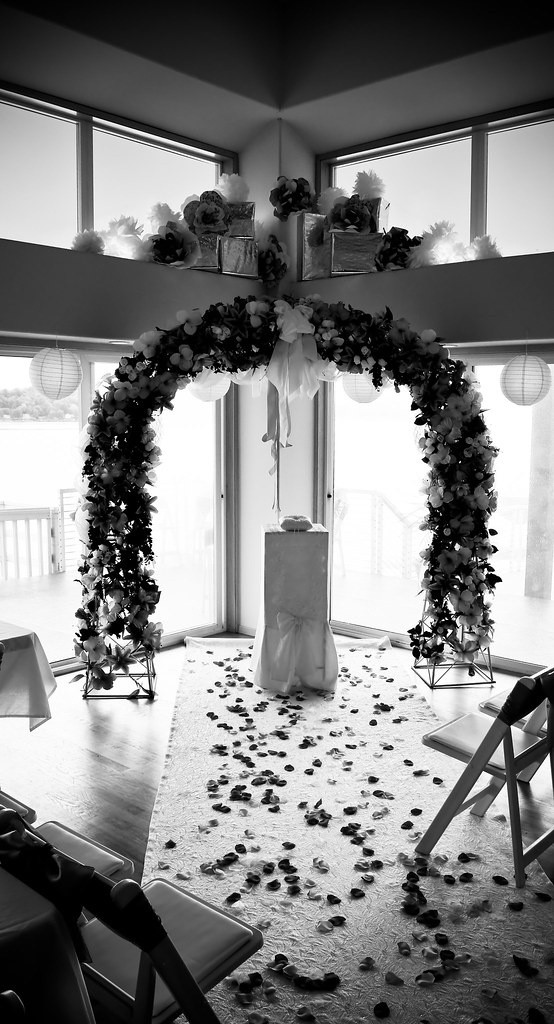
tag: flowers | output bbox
[72,170,504,272]
[72,292,505,699]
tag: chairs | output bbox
[415,664,554,889]
[0,820,135,931]
[0,806,265,1024]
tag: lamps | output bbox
[500,331,553,406]
[29,337,83,401]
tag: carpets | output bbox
[140,634,554,1024]
